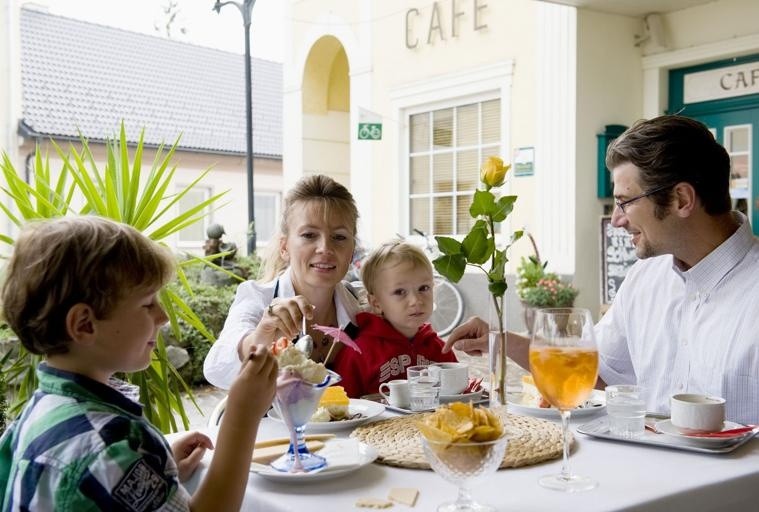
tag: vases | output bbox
[488,284,508,411]
[521,301,572,334]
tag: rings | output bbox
[267,301,277,315]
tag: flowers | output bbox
[431,156,523,284]
[516,233,579,309]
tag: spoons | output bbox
[293,315,314,359]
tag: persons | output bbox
[1,211,280,512]
[203,175,358,384]
[442,114,757,433]
[334,242,455,398]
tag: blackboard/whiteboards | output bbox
[602,216,641,303]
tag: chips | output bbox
[415,398,503,473]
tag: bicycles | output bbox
[353,227,465,338]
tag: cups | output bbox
[603,382,648,439]
[671,393,727,434]
[380,360,490,414]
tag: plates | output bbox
[484,383,613,417]
[266,398,388,431]
[573,416,758,455]
[251,438,377,483]
[655,416,749,442]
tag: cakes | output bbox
[521,376,551,408]
[312,386,350,421]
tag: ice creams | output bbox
[272,337,328,427]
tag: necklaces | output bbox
[308,321,338,362]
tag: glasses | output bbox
[615,184,675,213]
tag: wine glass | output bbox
[526,306,604,497]
[269,366,342,472]
[416,423,525,512]
